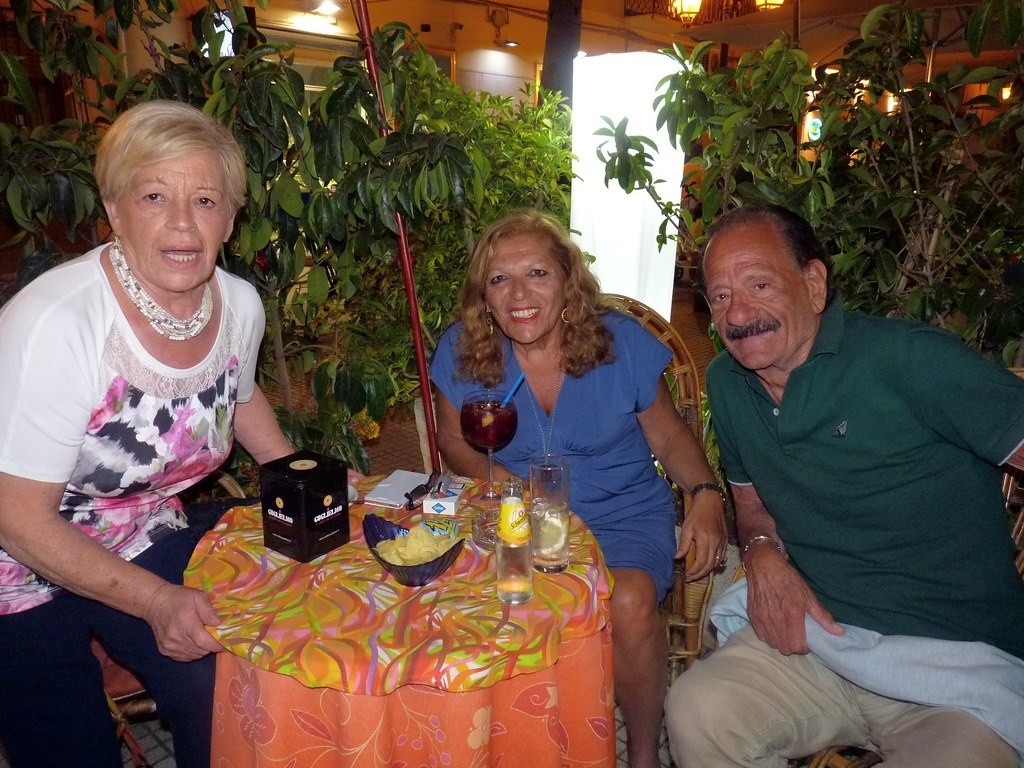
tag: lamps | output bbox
[674,0,703,29]
[756,0,785,10]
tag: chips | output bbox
[371,526,460,566]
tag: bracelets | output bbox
[692,482,727,505]
[741,534,782,573]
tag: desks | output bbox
[183,476,616,768]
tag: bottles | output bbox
[497,477,533,604]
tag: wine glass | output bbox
[460,390,518,511]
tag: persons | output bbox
[429,211,728,768]
[0,100,365,768]
[665,201,1024,768]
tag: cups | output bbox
[529,454,571,574]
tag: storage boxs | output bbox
[423,483,466,515]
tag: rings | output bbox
[716,555,724,562]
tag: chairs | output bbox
[732,368,1024,768]
[592,294,712,685]
[90,469,247,768]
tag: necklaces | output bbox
[512,346,564,470]
[109,237,214,341]
